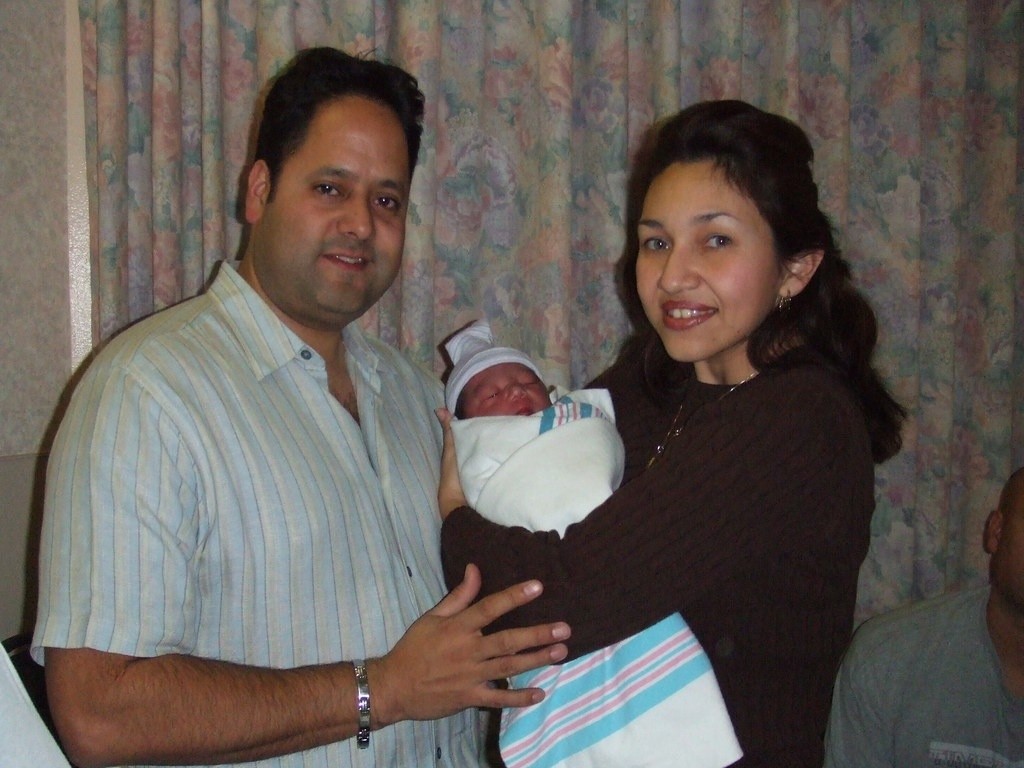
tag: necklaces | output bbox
[649,376,755,468]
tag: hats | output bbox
[439,316,543,420]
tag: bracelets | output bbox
[353,660,371,747]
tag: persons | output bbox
[28,47,571,768]
[439,99,907,768]
[824,467,1024,768]
[444,320,742,768]
